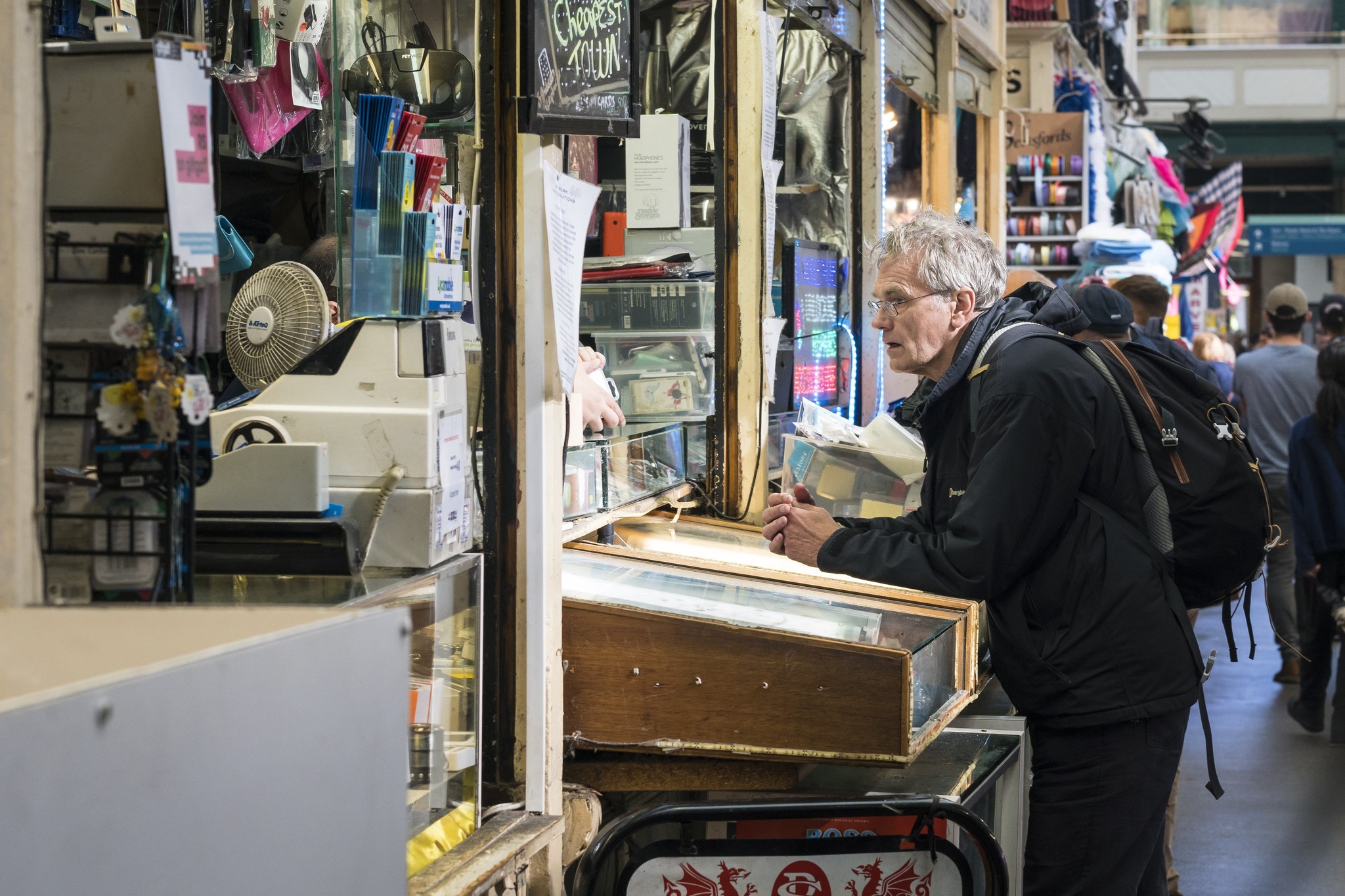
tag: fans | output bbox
[224,258,332,394]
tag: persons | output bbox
[1231,281,1331,684]
[1281,339,1345,742]
[1181,291,1345,396]
[765,208,1211,895]
[1108,274,1171,337]
[1062,282,1137,349]
[295,233,631,440]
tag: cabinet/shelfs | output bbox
[1006,106,1092,275]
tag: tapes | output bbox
[221,415,291,458]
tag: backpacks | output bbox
[969,321,1289,801]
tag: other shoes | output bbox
[1273,658,1301,682]
[1287,692,1325,732]
[1329,712,1345,745]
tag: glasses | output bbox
[867,288,957,317]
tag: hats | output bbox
[1264,282,1308,320]
[1071,283,1135,333]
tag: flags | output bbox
[1148,151,1191,206]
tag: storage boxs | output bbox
[558,534,980,767]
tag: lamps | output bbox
[1107,90,1215,151]
[1129,107,1220,169]
